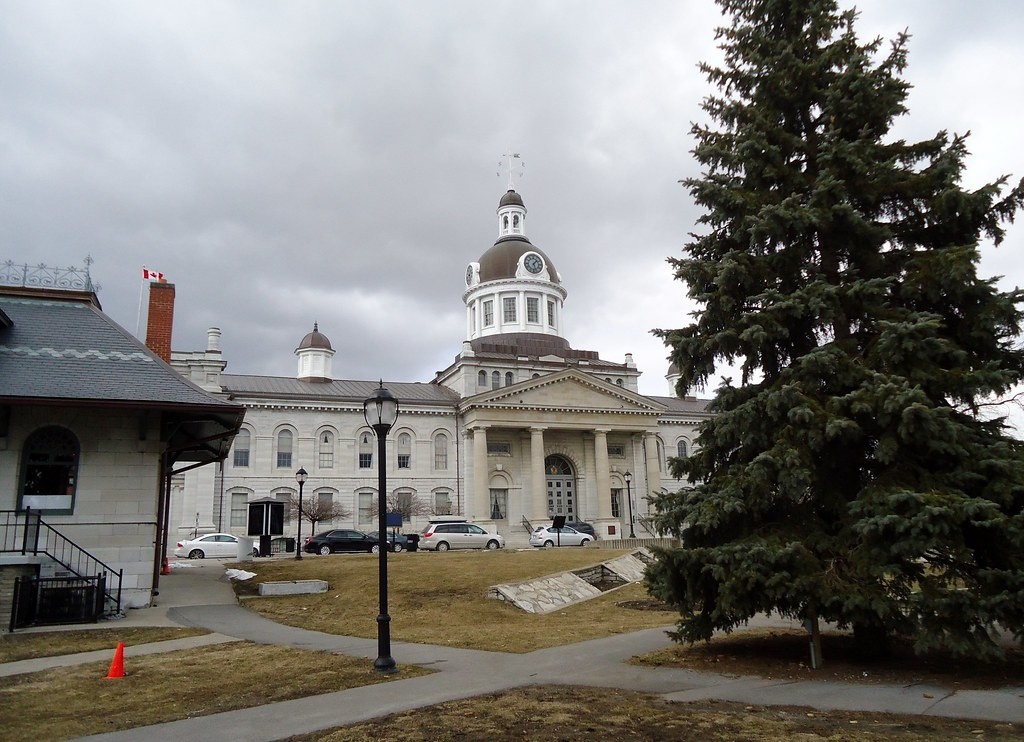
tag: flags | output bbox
[143,269,163,280]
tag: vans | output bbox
[417,523,506,552]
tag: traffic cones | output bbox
[102,642,127,679]
[163,557,170,574]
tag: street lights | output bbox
[363,378,400,672]
[294,466,308,561]
[623,469,637,538]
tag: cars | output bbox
[529,525,595,547]
[174,533,260,559]
[303,528,392,556]
[564,522,598,541]
[367,531,408,553]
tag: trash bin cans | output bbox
[406,534,419,552]
[285,538,295,553]
